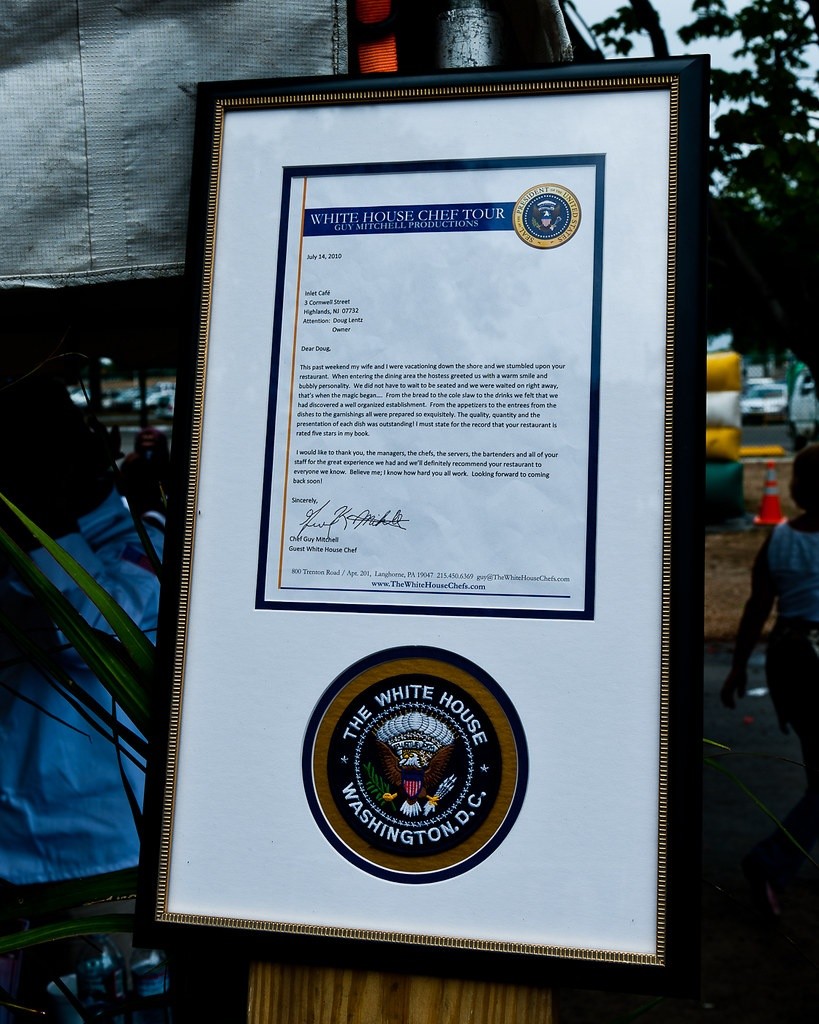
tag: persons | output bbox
[721,440,819,931]
[0,398,176,1024]
[114,423,175,523]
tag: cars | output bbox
[739,383,789,425]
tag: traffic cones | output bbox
[753,462,790,527]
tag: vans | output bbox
[787,371,819,450]
